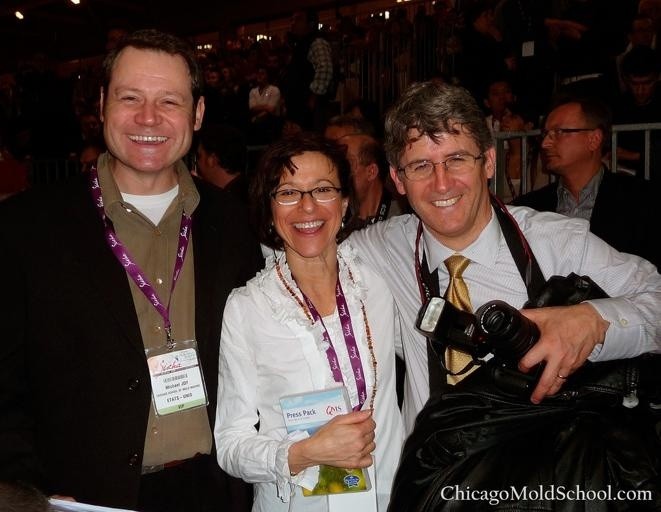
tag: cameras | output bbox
[411,292,550,397]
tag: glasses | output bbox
[541,128,594,139]
[397,154,484,181]
[269,186,342,206]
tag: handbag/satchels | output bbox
[387,273,661,512]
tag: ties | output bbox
[444,257,474,386]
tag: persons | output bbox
[1,3,661,511]
